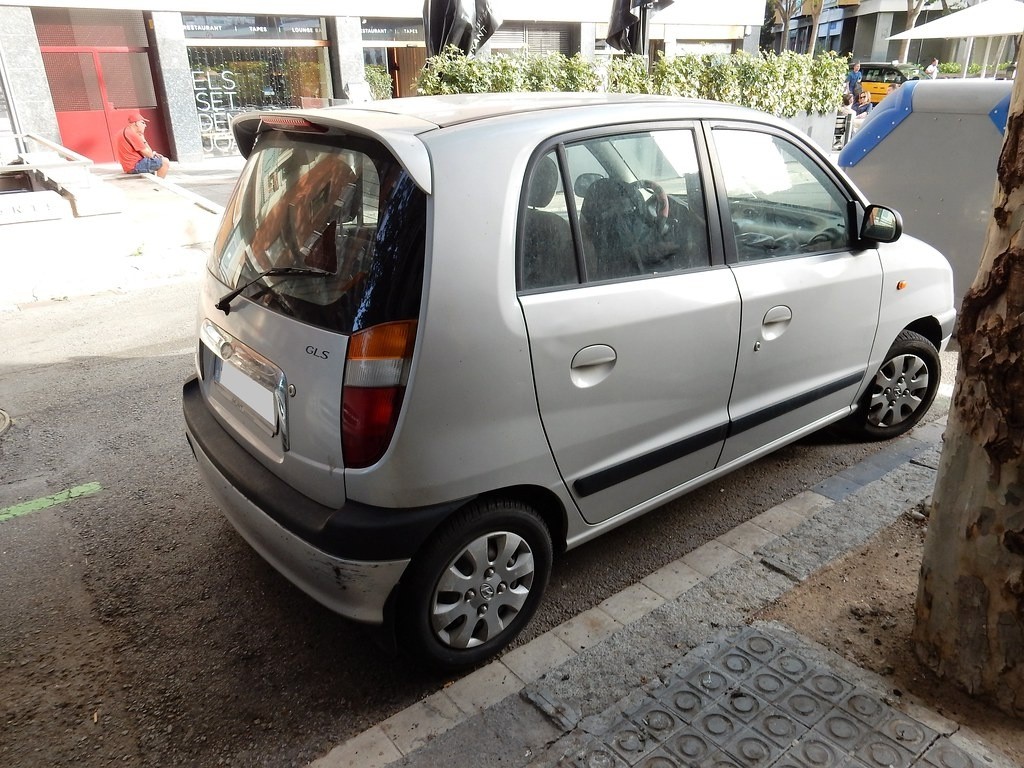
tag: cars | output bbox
[183,93,957,667]
[848,60,934,103]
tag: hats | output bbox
[127,113,150,123]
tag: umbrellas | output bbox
[884,0,1024,78]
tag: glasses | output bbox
[860,96,867,99]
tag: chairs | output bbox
[523,160,578,290]
[831,116,848,150]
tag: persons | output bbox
[887,84,897,95]
[117,113,170,179]
[925,57,938,80]
[844,63,862,96]
[851,91,872,119]
[885,72,895,83]
[834,94,856,151]
